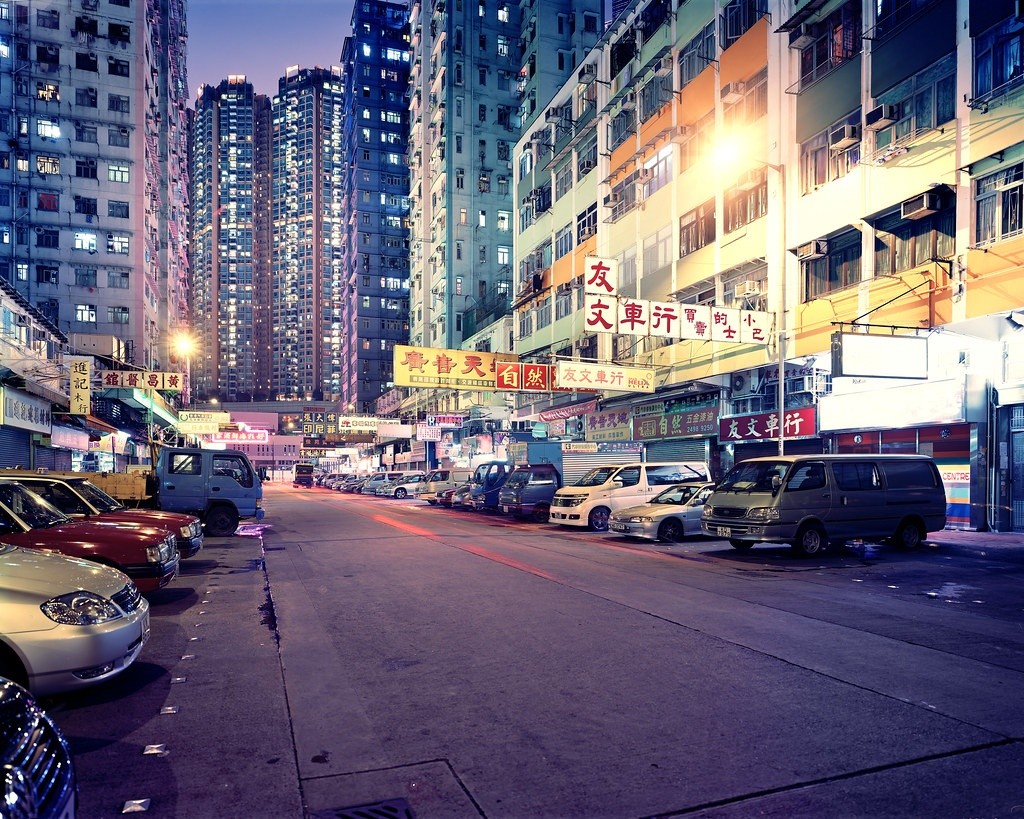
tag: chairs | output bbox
[761,470,780,489]
[799,470,822,489]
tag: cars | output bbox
[0,677,81,819]
[0,542,149,696]
[607,480,725,544]
[313,472,441,500]
[0,466,203,593]
[436,480,473,511]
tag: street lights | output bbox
[150,333,193,441]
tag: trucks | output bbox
[467,440,641,524]
[0,446,266,537]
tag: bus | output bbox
[292,463,313,489]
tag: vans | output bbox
[412,468,478,506]
[548,462,712,532]
[702,452,947,566]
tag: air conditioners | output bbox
[620,93,636,110]
[603,193,620,207]
[789,23,817,50]
[578,64,597,83]
[576,414,584,434]
[16,7,561,433]
[720,81,745,103]
[901,193,941,221]
[731,369,758,396]
[578,160,594,175]
[580,227,594,240]
[865,103,900,133]
[828,125,860,151]
[633,168,653,184]
[797,238,828,261]
[654,59,673,78]
[735,280,761,298]
[575,339,589,349]
[622,11,645,43]
[669,125,692,144]
